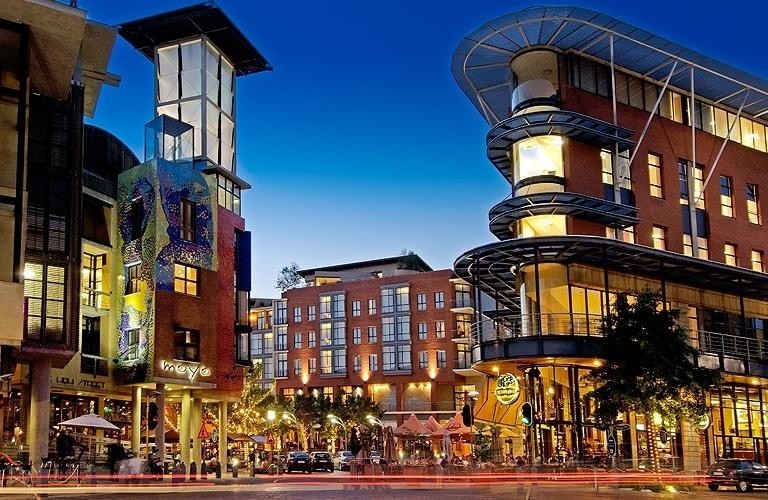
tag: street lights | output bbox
[467,390,480,455]
[327,413,348,452]
[267,409,276,432]
[282,411,300,453]
[366,414,386,458]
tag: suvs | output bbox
[706,457,768,493]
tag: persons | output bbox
[172,451,180,466]
[56,428,77,477]
[521,455,529,465]
[548,453,560,465]
[106,440,126,476]
[119,444,163,482]
[535,454,543,465]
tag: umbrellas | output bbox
[348,412,522,473]
[55,412,121,461]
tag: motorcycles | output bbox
[147,445,164,481]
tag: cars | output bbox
[272,449,382,474]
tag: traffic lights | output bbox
[521,402,533,427]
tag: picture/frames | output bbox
[716,418,725,433]
